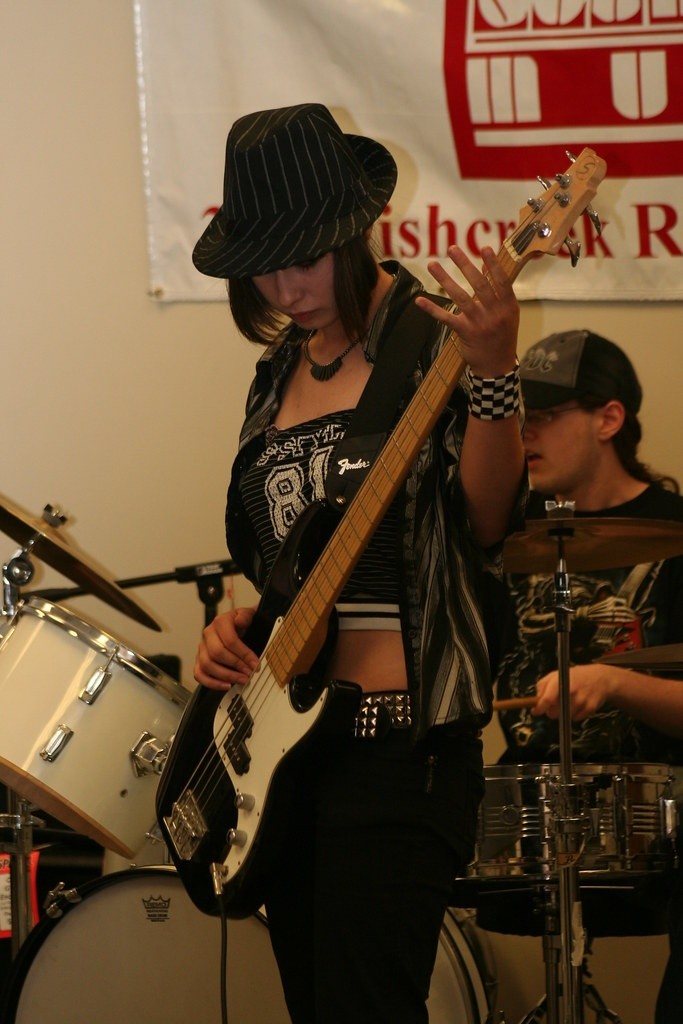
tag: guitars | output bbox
[153,137,611,923]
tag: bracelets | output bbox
[467,354,525,438]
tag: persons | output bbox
[496,328,683,1024]
[191,103,524,1024]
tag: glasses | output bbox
[527,405,586,427]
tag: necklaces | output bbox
[304,323,370,381]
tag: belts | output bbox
[328,691,415,738]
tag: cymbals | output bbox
[591,642,682,667]
[501,512,683,576]
[1,491,165,634]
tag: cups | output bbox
[0,812,47,939]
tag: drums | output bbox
[9,865,496,1024]
[457,761,683,899]
[0,594,195,863]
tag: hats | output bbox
[520,329,642,412]
[193,103,398,279]
